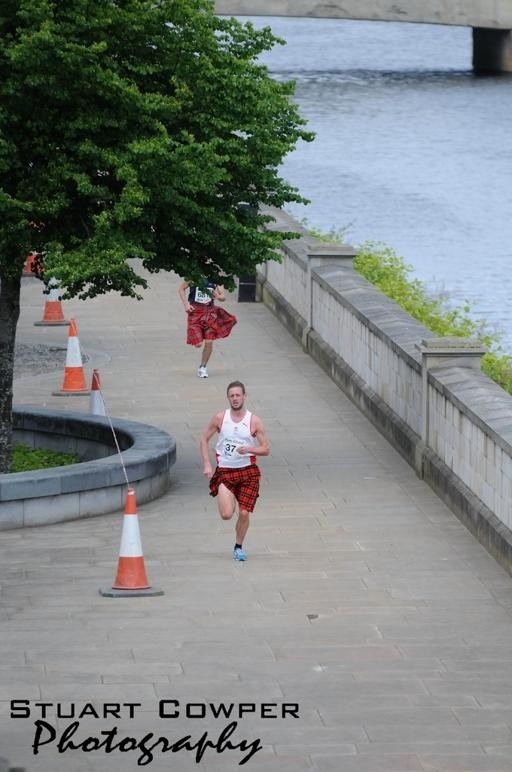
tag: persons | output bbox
[179,256,238,378]
[200,381,270,561]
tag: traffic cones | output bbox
[51,318,90,396]
[22,251,45,276]
[90,368,108,419]
[100,487,166,599]
[34,276,70,326]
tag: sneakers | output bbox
[197,367,209,378]
[233,546,248,561]
